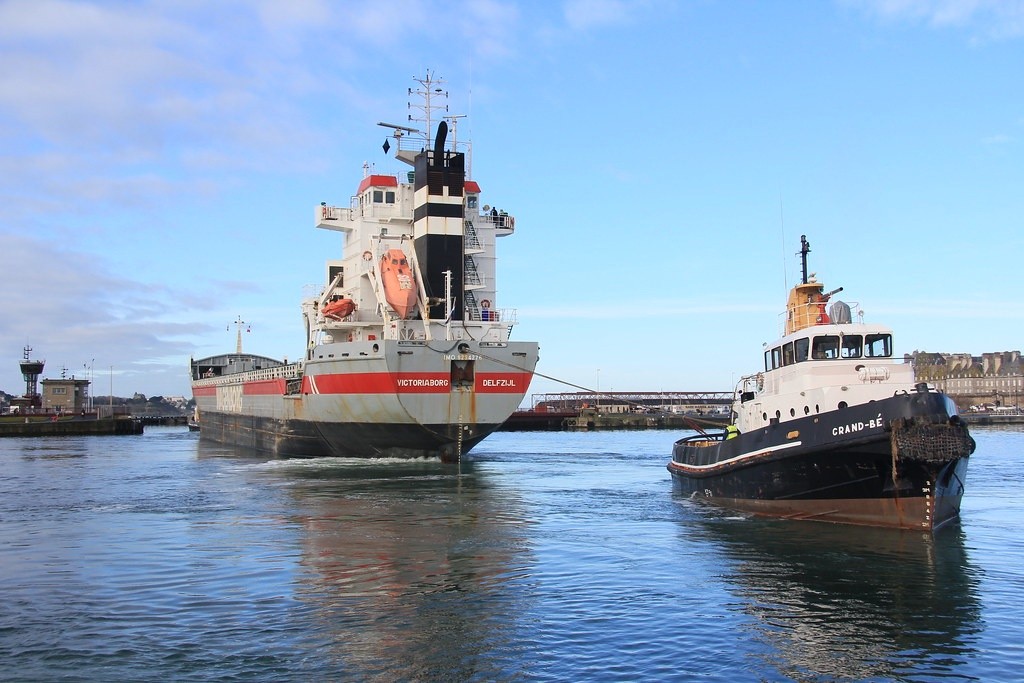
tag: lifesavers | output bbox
[362,250,373,261]
[755,371,764,392]
[481,299,490,310]
[569,421,576,425]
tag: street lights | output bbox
[596,368,601,406]
[109,365,114,406]
[91,359,95,412]
[83,363,87,411]
[610,387,613,413]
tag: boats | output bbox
[188,416,200,431]
[320,297,355,322]
[188,68,542,466]
[378,249,418,320]
[664,198,976,533]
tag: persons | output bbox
[722,421,741,441]
[490,207,498,222]
[499,209,508,226]
